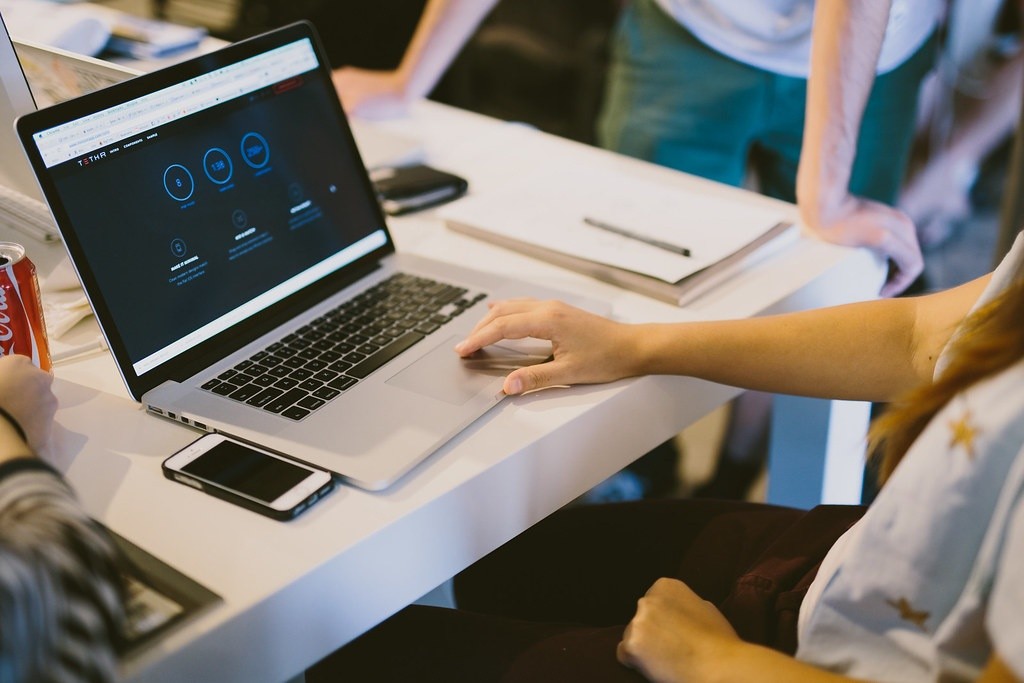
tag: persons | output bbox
[455,91,1024,683]
[327,0,968,512]
[0,352,128,683]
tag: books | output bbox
[366,164,469,217]
[442,164,800,306]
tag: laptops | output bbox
[15,27,607,490]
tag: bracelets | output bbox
[1,407,28,444]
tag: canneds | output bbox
[0,241,53,375]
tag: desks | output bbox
[0,2,901,683]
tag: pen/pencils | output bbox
[584,216,691,258]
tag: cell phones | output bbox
[162,431,333,520]
